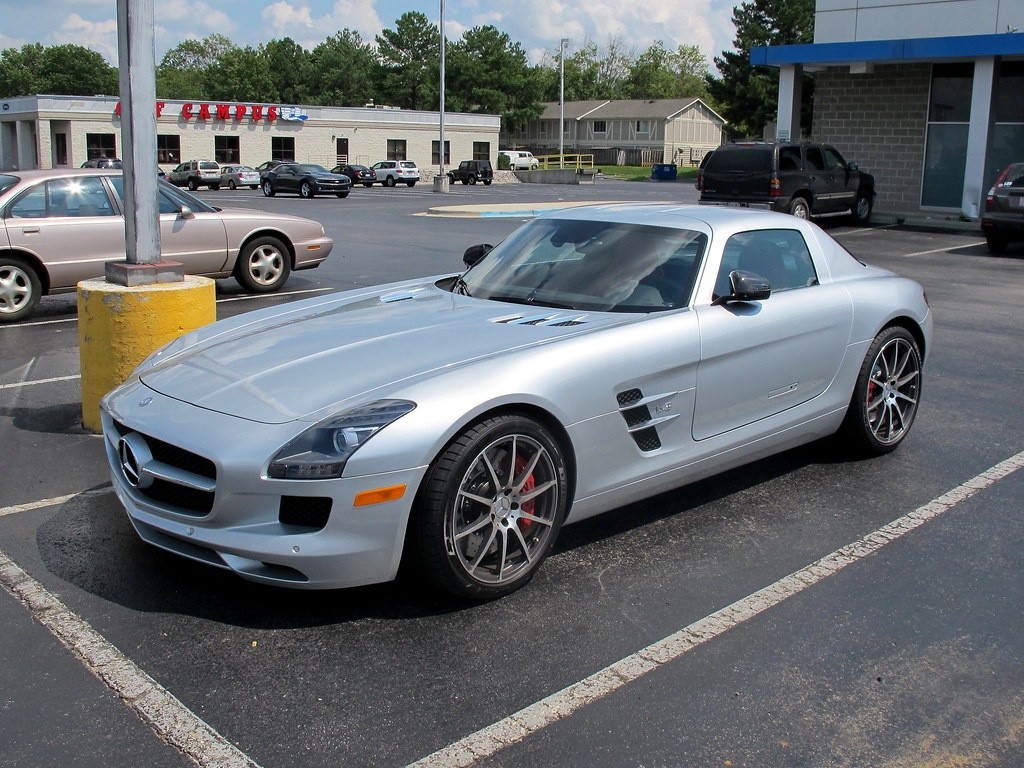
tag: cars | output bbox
[981,163,1024,255]
[260,163,352,199]
[158,166,174,186]
[208,164,260,190]
[80,157,122,169]
[254,158,300,187]
[0,168,335,324]
[328,164,377,188]
[695,150,714,190]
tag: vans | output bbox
[499,150,540,170]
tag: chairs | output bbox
[613,230,665,289]
[50,184,70,217]
[737,240,790,290]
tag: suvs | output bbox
[370,160,421,187]
[446,160,494,185]
[698,136,877,227]
[166,159,222,191]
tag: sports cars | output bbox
[97,199,933,609]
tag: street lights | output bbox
[560,39,571,168]
